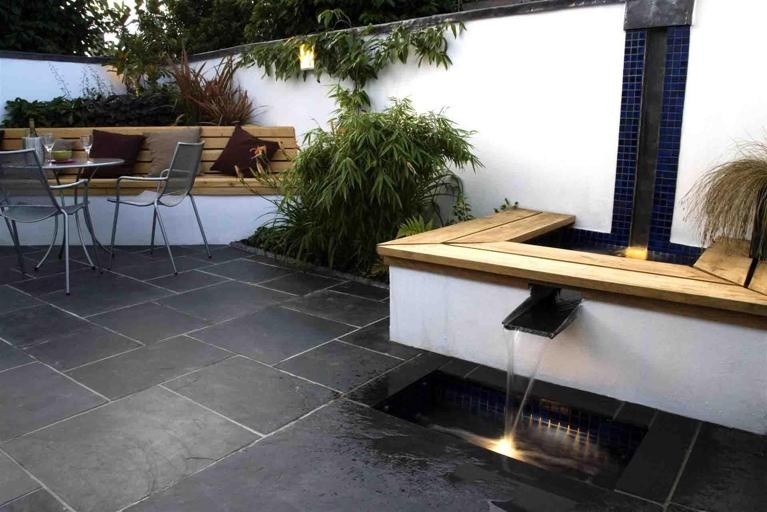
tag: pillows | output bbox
[84,130,148,178]
[145,127,203,176]
[0,130,5,149]
[210,126,280,176]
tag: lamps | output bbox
[299,43,315,70]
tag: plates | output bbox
[51,160,76,164]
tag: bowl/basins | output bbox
[52,150,72,161]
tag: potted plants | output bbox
[679,140,767,261]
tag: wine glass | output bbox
[80,135,96,164]
[40,133,55,166]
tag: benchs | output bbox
[0,126,298,196]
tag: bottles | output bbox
[28,118,38,137]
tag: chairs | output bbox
[0,148,94,295]
[108,140,212,275]
